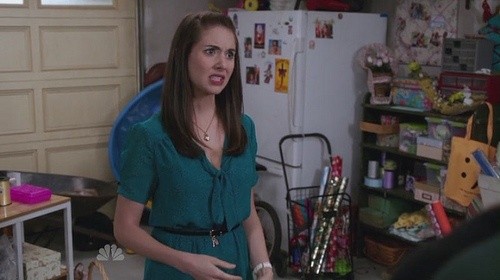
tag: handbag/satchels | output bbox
[443,102,498,209]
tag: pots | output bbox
[0,170,120,220]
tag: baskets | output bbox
[362,233,413,268]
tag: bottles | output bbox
[365,148,406,189]
[0,170,11,207]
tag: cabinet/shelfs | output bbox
[361,103,475,245]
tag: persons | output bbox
[112,11,274,280]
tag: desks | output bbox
[0,191,74,280]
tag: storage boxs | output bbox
[425,116,466,142]
[440,171,466,213]
[398,123,425,153]
[413,181,440,204]
[23,242,62,280]
[423,162,445,186]
[415,137,450,160]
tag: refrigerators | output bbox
[226,9,389,258]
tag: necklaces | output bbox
[194,109,217,142]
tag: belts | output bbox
[154,222,243,238]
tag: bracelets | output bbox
[253,262,271,280]
